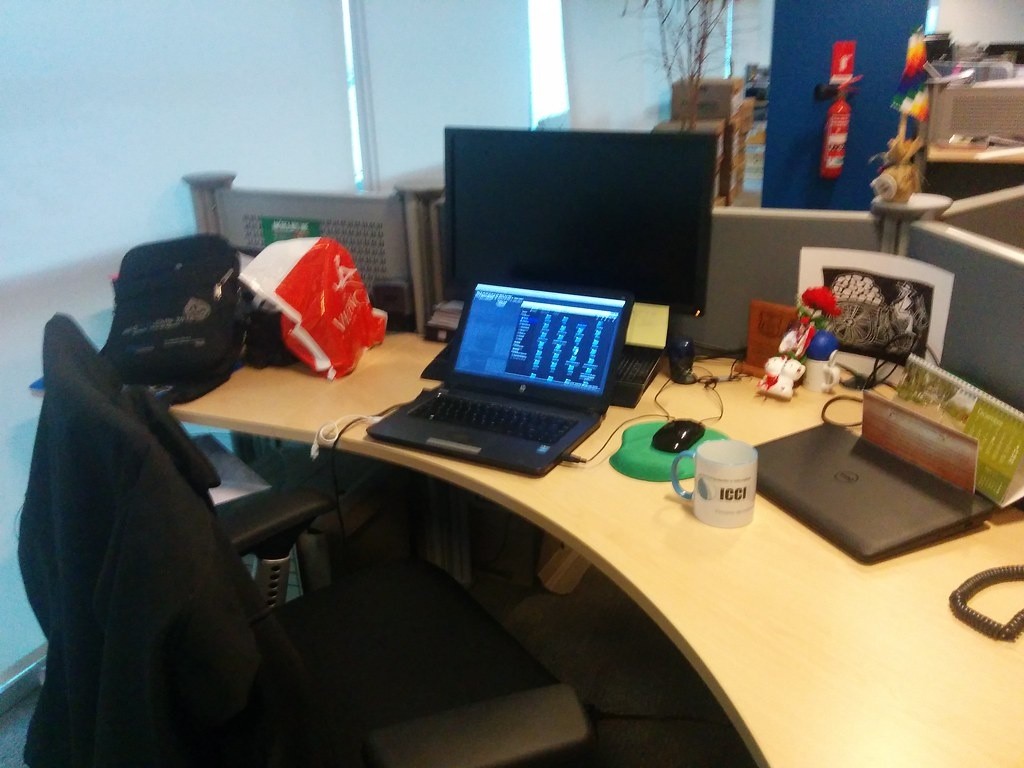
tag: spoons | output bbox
[828,349,838,393]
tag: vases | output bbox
[755,322,817,399]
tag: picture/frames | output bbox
[799,246,955,390]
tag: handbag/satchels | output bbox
[238,236,388,379]
[98,232,247,404]
[245,307,302,370]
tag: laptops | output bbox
[365,279,636,476]
[754,422,995,563]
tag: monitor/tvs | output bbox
[444,127,718,317]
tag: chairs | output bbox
[21,317,597,768]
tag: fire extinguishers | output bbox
[814,74,865,184]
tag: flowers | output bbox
[794,284,844,331]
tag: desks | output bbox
[27,320,1024,768]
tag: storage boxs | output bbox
[713,174,722,198]
[740,99,755,134]
[653,119,726,166]
[726,114,746,167]
[714,195,727,209]
[721,160,745,206]
[671,77,746,117]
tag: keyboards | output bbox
[421,333,661,408]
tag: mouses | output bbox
[653,419,706,454]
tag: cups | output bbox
[672,438,759,528]
[802,358,841,393]
